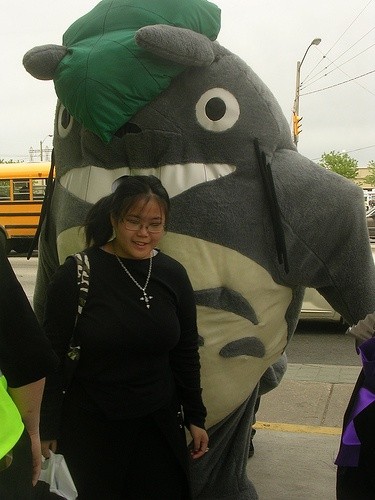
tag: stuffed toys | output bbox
[23,0,375,500]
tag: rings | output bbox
[204,447,209,453]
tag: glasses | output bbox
[118,215,167,233]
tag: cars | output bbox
[297,243,375,330]
[365,207,375,239]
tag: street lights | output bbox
[291,37,322,147]
[40,134,53,161]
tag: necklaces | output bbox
[109,239,154,308]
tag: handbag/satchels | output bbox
[37,449,79,500]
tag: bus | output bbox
[0,161,56,256]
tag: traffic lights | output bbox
[293,114,303,136]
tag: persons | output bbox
[1,225,52,500]
[45,174,210,500]
